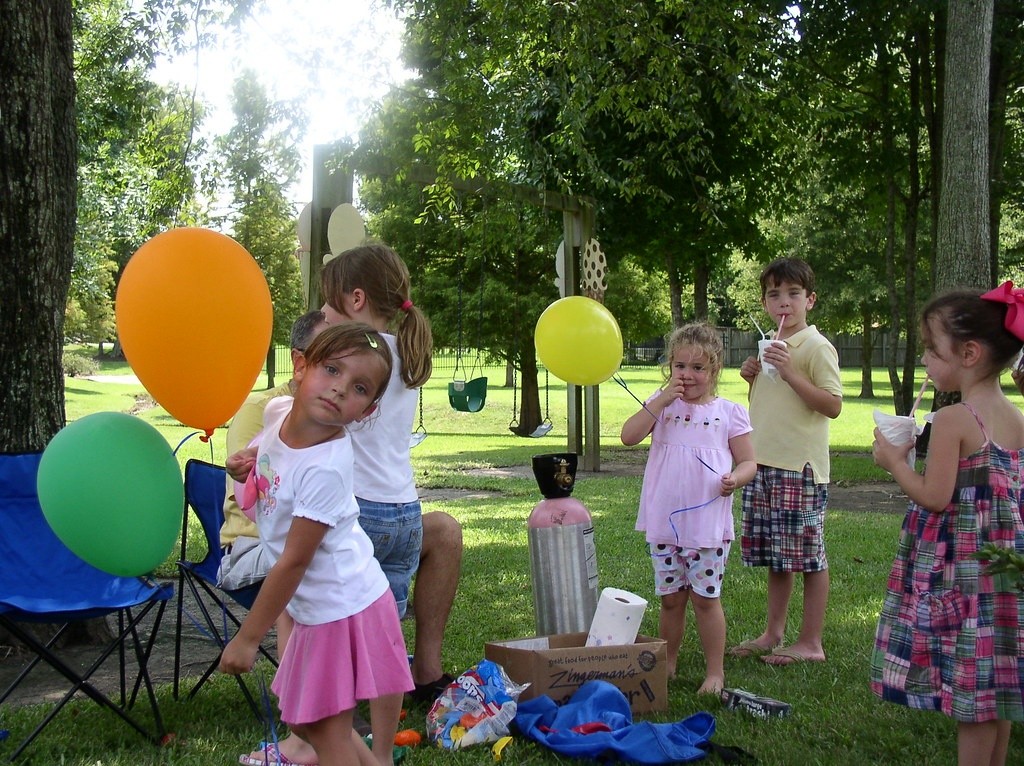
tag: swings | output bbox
[508,201,554,438]
[447,184,488,413]
[381,173,429,451]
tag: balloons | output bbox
[532,296,624,386]
[37,411,185,578]
[116,227,273,442]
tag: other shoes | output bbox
[409,672,453,700]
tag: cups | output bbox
[758,339,787,374]
[874,411,916,476]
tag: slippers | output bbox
[726,639,768,659]
[239,743,319,766]
[758,646,826,667]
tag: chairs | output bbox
[175,459,280,723]
[0,452,174,762]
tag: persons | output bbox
[728,254,842,666]
[217,238,463,766]
[870,279,1024,766]
[621,316,757,697]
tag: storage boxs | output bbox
[484,631,668,715]
[720,688,790,720]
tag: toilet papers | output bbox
[585,585,648,645]
